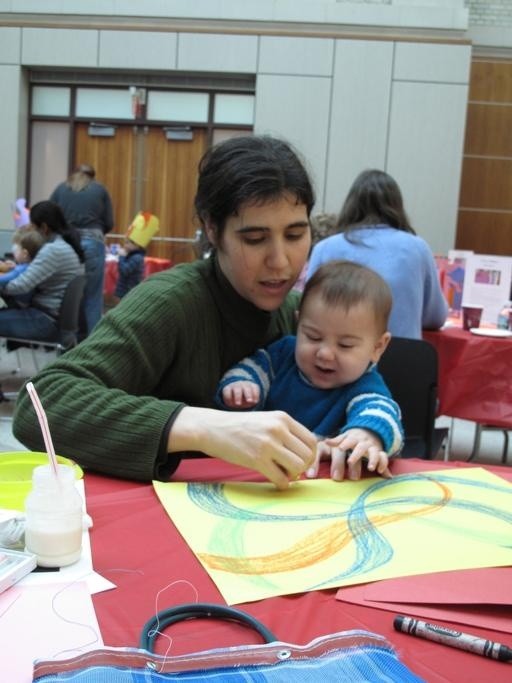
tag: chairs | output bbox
[377,334,454,464]
[0,273,89,403]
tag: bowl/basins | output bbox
[1,449,83,513]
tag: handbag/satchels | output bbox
[27,599,431,683]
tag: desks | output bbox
[0,440,512,681]
[421,320,512,464]
[102,251,172,301]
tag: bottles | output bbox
[497,301,512,330]
[22,466,85,568]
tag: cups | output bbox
[461,303,483,331]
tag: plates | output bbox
[469,326,511,338]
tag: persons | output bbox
[1,163,146,352]
[17,135,405,491]
[306,168,449,460]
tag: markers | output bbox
[393,616,511,660]
